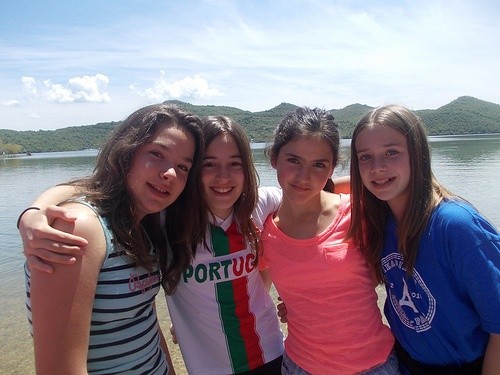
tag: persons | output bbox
[18,104,399,374]
[277,103,500,374]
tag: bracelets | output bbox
[17,207,40,229]
